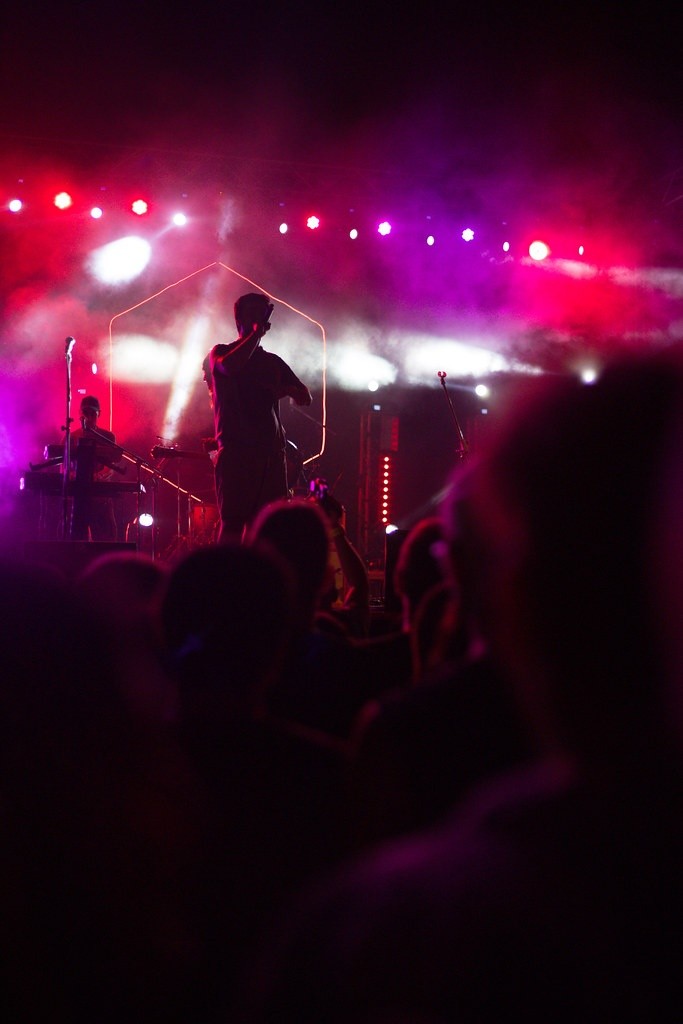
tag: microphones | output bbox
[65,336,76,354]
[262,304,275,325]
[79,416,87,429]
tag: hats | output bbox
[81,394,100,411]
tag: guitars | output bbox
[151,444,211,460]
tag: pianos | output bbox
[25,443,145,552]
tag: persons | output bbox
[0,340,683,1024]
[61,396,123,543]
[204,292,314,543]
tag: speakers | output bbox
[382,530,412,611]
[20,539,138,578]
